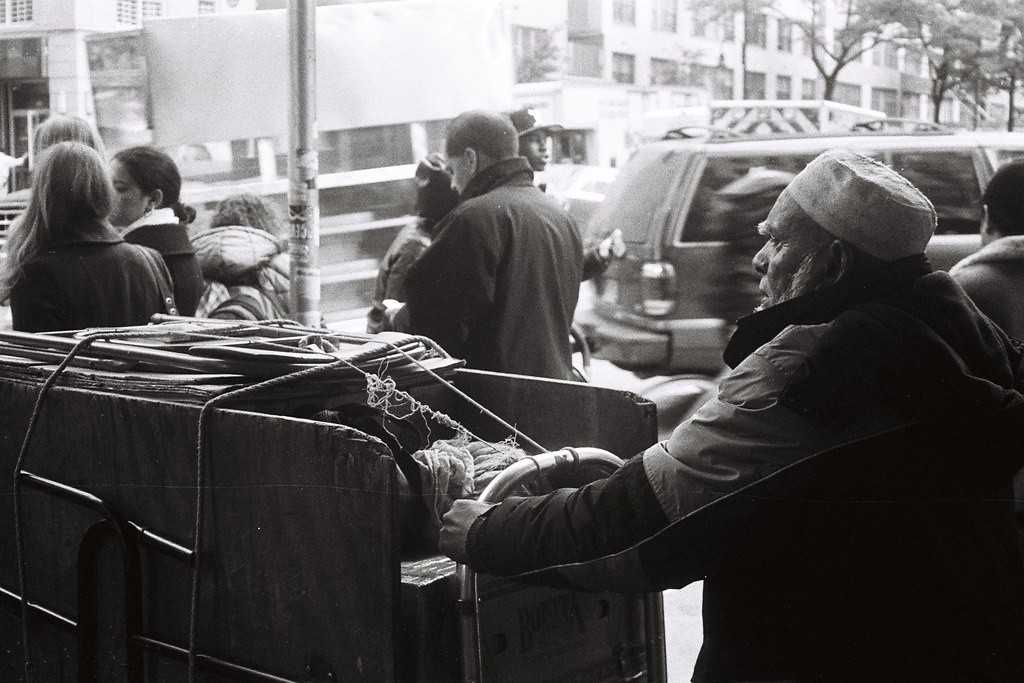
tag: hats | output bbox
[507,104,564,139]
[789,149,937,263]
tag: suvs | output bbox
[573,119,1024,379]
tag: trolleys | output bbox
[428,445,666,683]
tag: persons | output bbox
[946,158,1024,341]
[367,105,626,382]
[438,147,1024,683]
[0,115,327,330]
